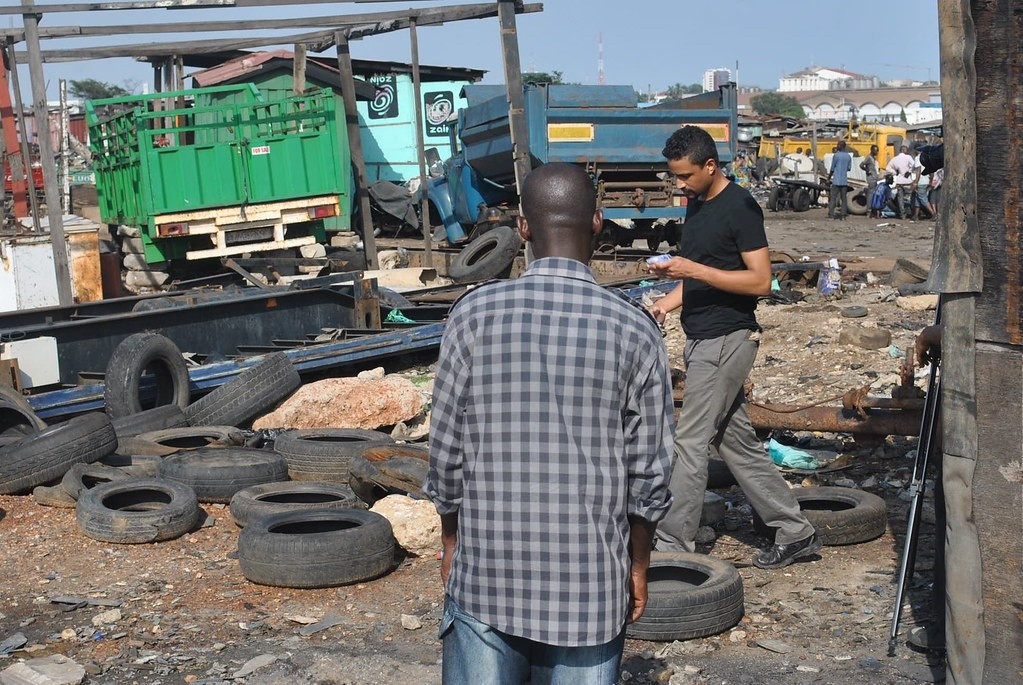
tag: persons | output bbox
[785,141,943,222]
[636,125,819,570]
[417,161,678,685]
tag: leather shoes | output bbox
[752,531,823,570]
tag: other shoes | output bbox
[840,215,846,221]
[825,215,834,220]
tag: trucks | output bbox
[416,72,738,259]
[84,86,358,281]
[759,122,907,199]
